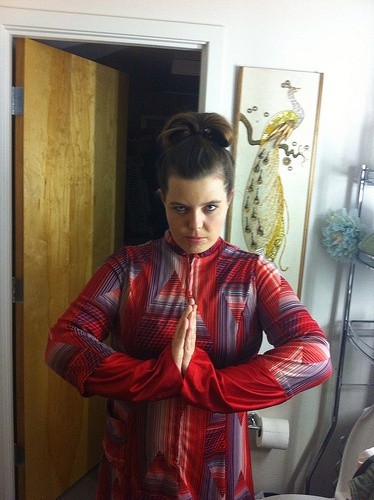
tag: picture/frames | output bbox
[227,67,323,302]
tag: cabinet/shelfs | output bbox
[306,166,374,495]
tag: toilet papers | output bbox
[256,416,290,450]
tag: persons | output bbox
[43,112,334,500]
